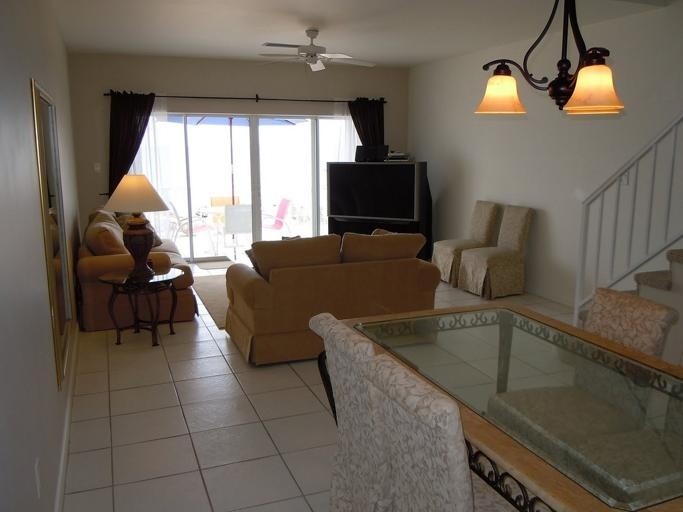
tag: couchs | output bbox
[224,258,441,367]
[76,210,198,331]
[246,229,426,278]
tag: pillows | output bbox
[85,210,129,255]
[114,214,163,246]
[87,205,118,222]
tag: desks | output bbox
[97,267,185,346]
[316,302,683,512]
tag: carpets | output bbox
[194,260,237,270]
[193,273,233,329]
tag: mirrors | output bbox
[31,76,77,392]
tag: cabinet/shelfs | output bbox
[328,216,433,263]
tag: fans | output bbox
[257,29,352,72]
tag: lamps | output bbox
[473,0,625,116]
[103,174,170,279]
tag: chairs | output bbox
[564,364,683,499]
[309,311,549,511]
[487,288,681,461]
[168,198,290,260]
[430,200,504,287]
[458,205,535,299]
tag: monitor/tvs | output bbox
[327,162,428,225]
